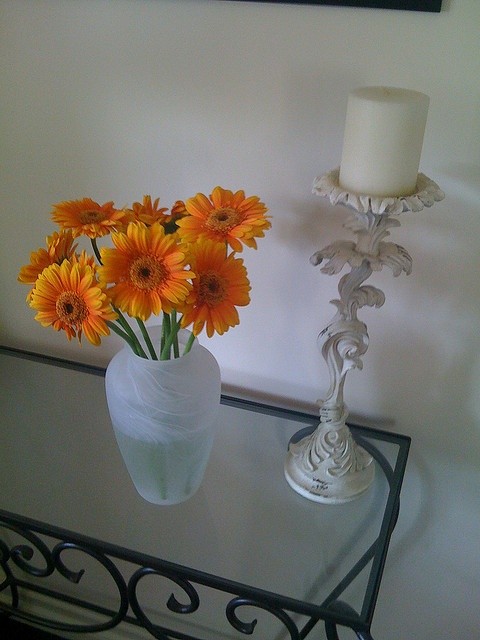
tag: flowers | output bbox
[16,186,272,362]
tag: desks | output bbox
[0,344,412,640]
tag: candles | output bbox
[338,85,431,197]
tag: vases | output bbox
[105,324,222,507]
